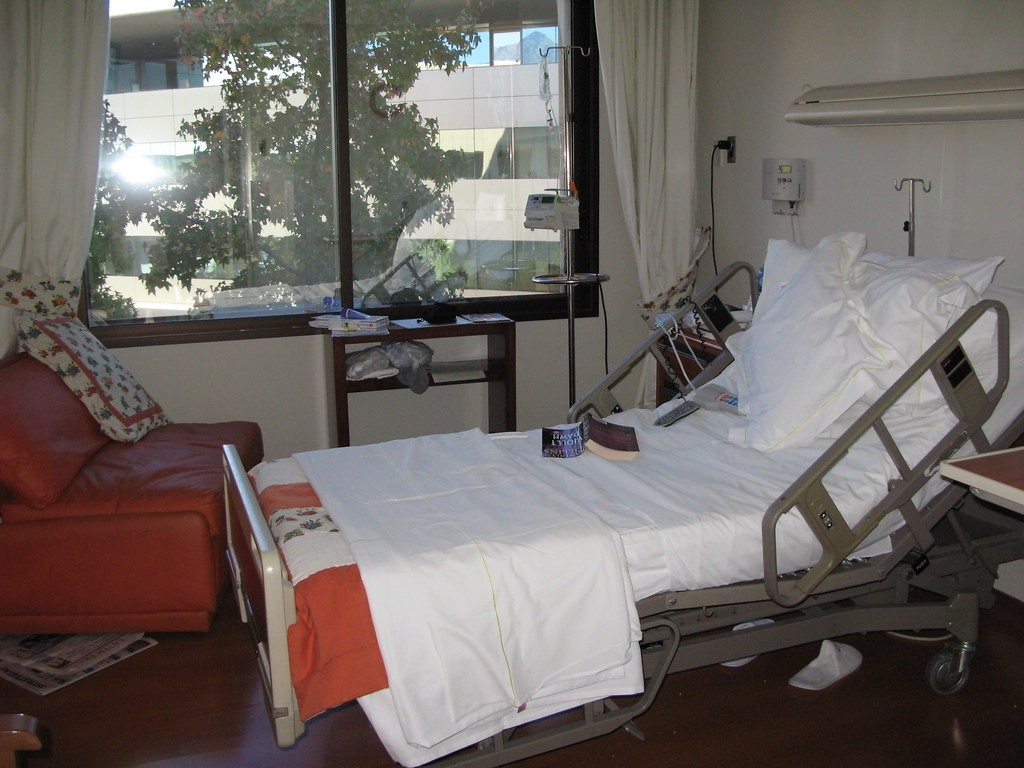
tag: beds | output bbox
[221,262,1024,768]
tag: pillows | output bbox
[0,353,105,508]
[749,240,1004,302]
[18,311,173,444]
[726,233,905,453]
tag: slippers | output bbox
[789,640,863,691]
[720,618,775,668]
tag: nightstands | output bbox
[653,323,730,411]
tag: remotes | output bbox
[655,401,700,427]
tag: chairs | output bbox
[0,288,265,633]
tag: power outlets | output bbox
[726,136,737,164]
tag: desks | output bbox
[331,313,516,447]
[938,449,1023,514]
